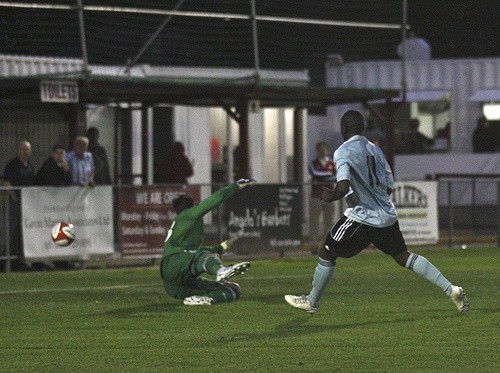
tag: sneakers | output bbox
[183,295,215,306]
[284,294,320,314]
[216,262,251,282]
[450,285,470,315]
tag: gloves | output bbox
[220,240,232,254]
[237,177,257,190]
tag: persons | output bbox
[283,110,472,315]
[160,176,253,306]
[2,142,36,271]
[395,118,434,157]
[67,137,97,187]
[85,127,112,186]
[309,142,337,253]
[164,142,192,187]
[30,146,73,271]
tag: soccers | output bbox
[50,222,76,247]
